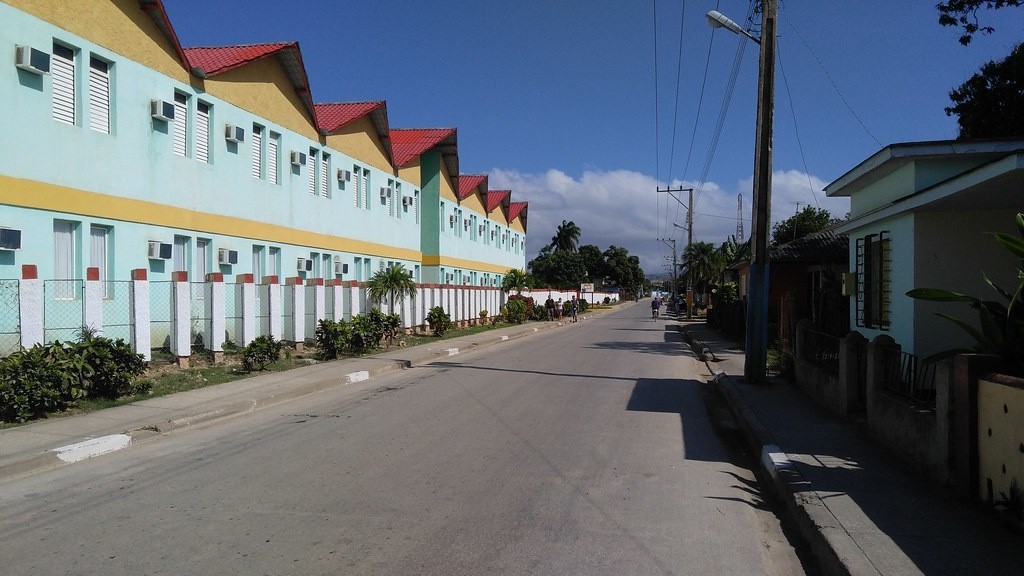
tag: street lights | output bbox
[706,9,777,388]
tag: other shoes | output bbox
[573,320,577,323]
[652,317,654,319]
[558,319,562,321]
[547,319,554,321]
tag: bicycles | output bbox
[652,309,658,322]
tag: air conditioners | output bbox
[463,275,471,283]
[465,219,472,227]
[147,240,173,261]
[14,45,52,77]
[479,225,527,248]
[0,227,22,251]
[290,150,308,166]
[297,258,313,272]
[403,196,413,206]
[380,185,392,198]
[446,273,454,281]
[449,213,458,224]
[480,277,497,284]
[338,169,351,182]
[381,267,391,275]
[151,99,176,123]
[218,247,239,265]
[404,269,415,279]
[336,262,349,275]
[225,124,245,145]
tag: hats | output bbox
[654,297,658,299]
[572,295,575,297]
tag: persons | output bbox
[555,298,566,320]
[569,295,579,323]
[650,294,687,318]
[543,295,557,321]
